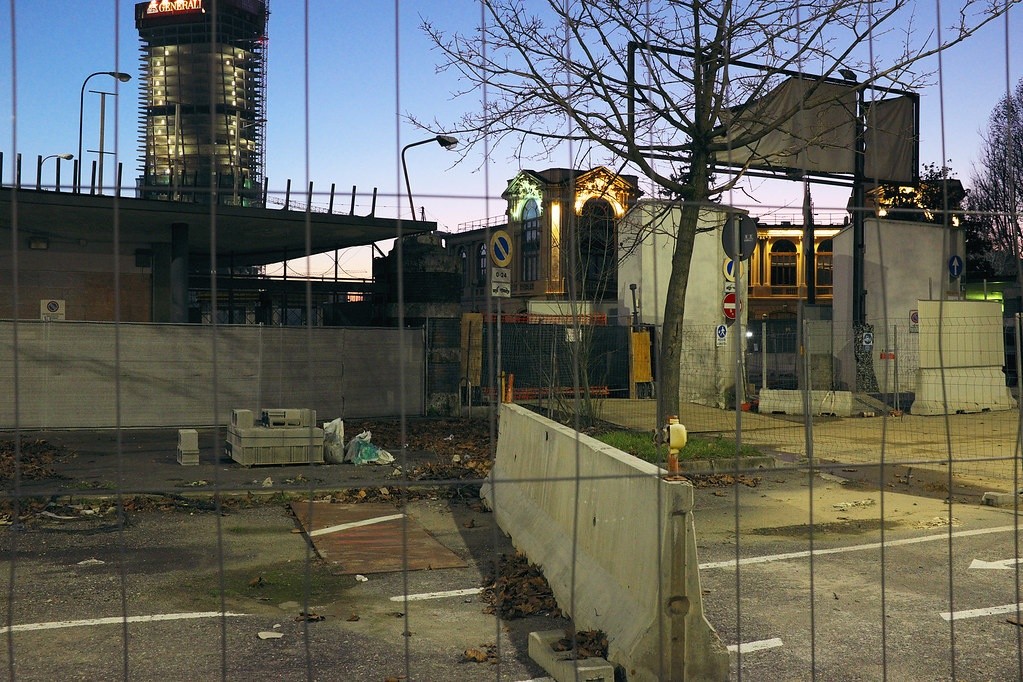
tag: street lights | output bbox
[78,71,133,194]
[838,69,867,324]
[401,135,460,225]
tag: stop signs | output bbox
[721,293,743,320]
